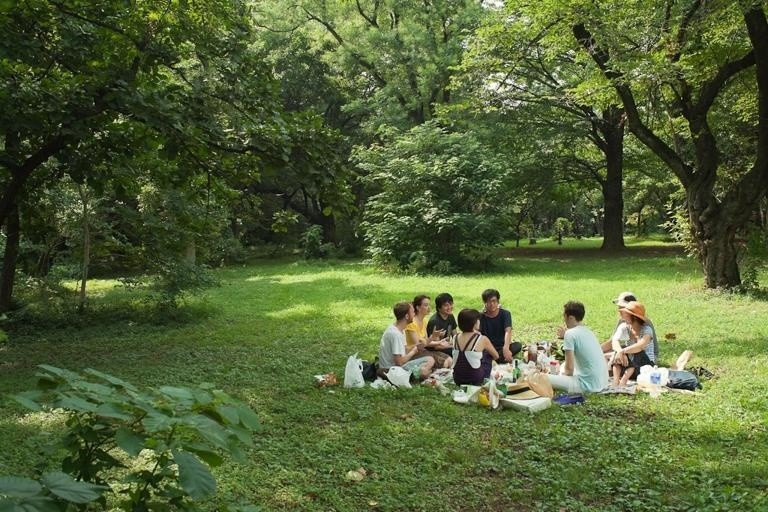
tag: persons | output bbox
[378,289,522,387]
[600,292,659,388]
[541,300,609,394]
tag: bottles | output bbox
[512,360,520,383]
[650,365,661,398]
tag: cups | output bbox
[528,346,537,363]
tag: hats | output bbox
[619,301,646,322]
[505,381,541,400]
[613,291,637,307]
